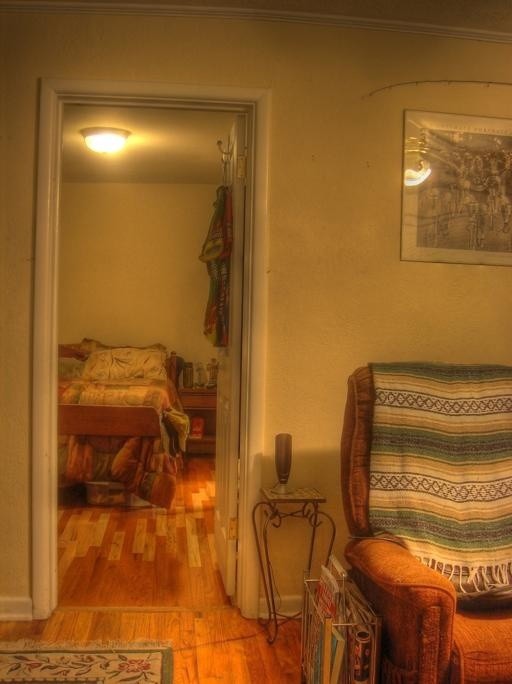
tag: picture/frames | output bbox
[400,109,512,268]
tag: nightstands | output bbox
[178,388,219,454]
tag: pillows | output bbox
[57,347,169,385]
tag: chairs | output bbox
[340,360,512,684]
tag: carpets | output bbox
[0,637,174,684]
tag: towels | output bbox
[198,184,234,348]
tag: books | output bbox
[301,553,378,684]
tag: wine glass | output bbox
[270,433,295,497]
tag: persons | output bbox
[415,145,512,252]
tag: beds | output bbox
[57,337,190,510]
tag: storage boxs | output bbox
[85,482,127,505]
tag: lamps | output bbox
[79,126,131,153]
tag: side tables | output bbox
[250,487,336,645]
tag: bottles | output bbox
[182,361,194,389]
